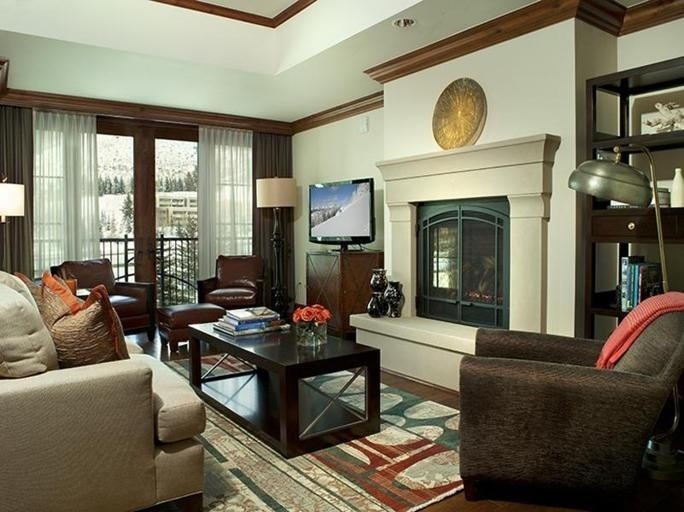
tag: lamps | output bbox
[0,171,25,224]
[256,173,296,314]
[568,142,670,294]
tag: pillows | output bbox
[1,269,128,381]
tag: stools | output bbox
[157,301,225,353]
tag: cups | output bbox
[670,167,684,208]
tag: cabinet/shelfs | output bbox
[584,53,683,341]
[304,249,384,339]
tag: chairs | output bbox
[196,254,262,308]
[51,257,156,342]
[458,311,684,501]
[0,343,205,511]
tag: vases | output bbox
[367,267,403,319]
[297,321,328,348]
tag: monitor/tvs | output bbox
[308,178,375,251]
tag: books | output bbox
[212,306,289,339]
[619,255,661,314]
[605,186,673,210]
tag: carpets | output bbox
[162,355,464,509]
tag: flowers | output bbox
[291,301,331,340]
